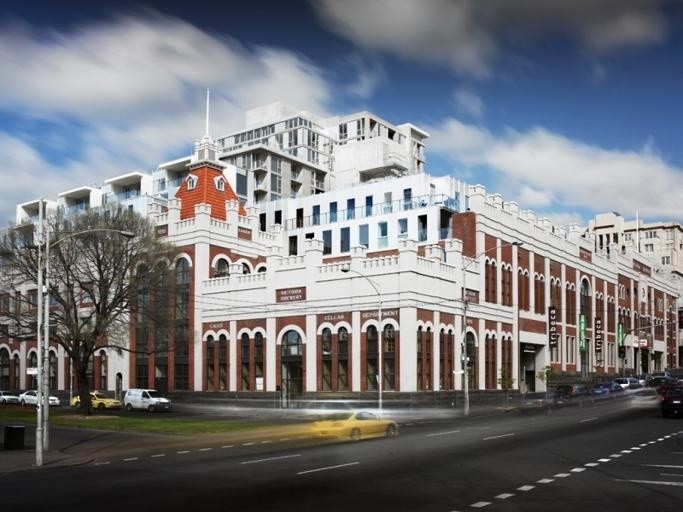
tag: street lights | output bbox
[341,269,382,419]
[35,196,136,468]
[462,241,524,416]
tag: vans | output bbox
[124,388,172,413]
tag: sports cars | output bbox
[309,409,400,443]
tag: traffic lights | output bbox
[618,346,626,358]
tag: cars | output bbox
[18,390,61,407]
[70,390,123,411]
[0,390,19,405]
[518,368,683,419]
